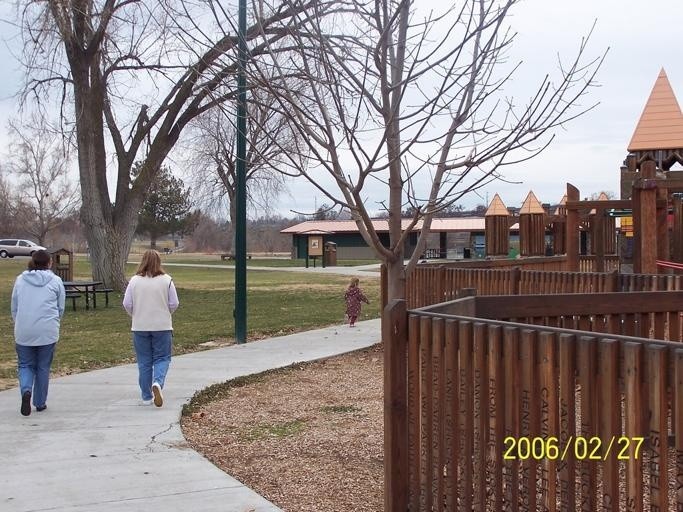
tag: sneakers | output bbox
[19,389,32,416]
[36,403,47,411]
[152,382,164,408]
[142,397,154,405]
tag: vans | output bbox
[0,238,46,259]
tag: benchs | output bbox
[66,289,114,308]
[67,293,81,311]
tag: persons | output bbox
[345,278,370,327]
[11,250,66,416]
[123,250,179,407]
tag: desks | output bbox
[62,282,102,310]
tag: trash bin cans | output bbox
[323,241,337,266]
[464,247,470,258]
[544,244,554,255]
[51,248,74,283]
